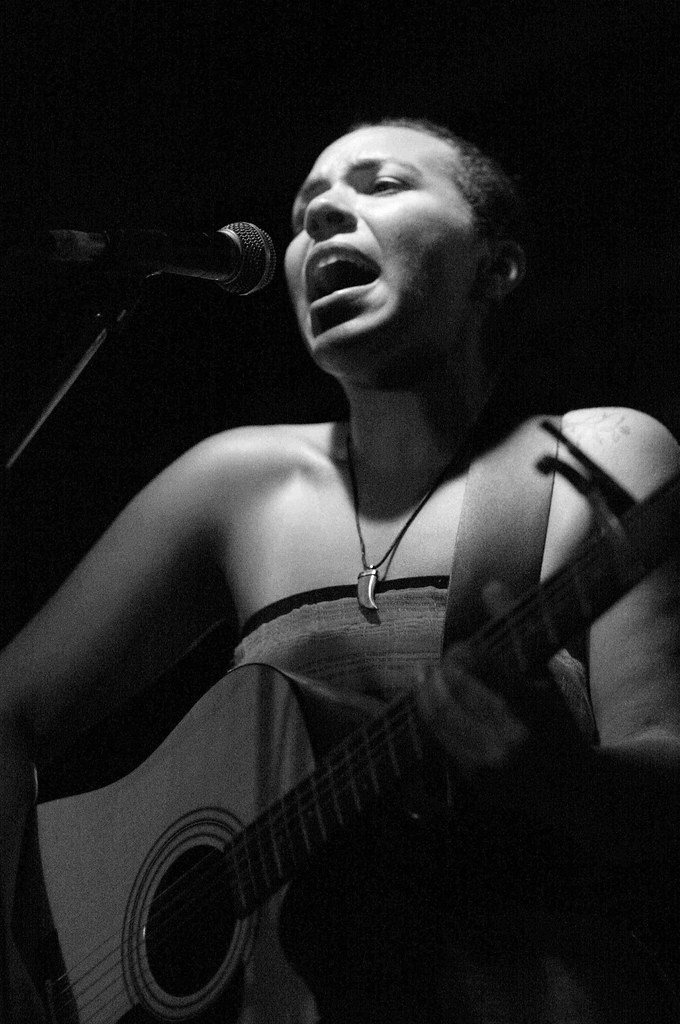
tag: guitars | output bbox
[0,465,680,1024]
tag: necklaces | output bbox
[345,428,467,610]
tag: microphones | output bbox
[44,220,279,295]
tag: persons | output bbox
[0,118,680,1024]
